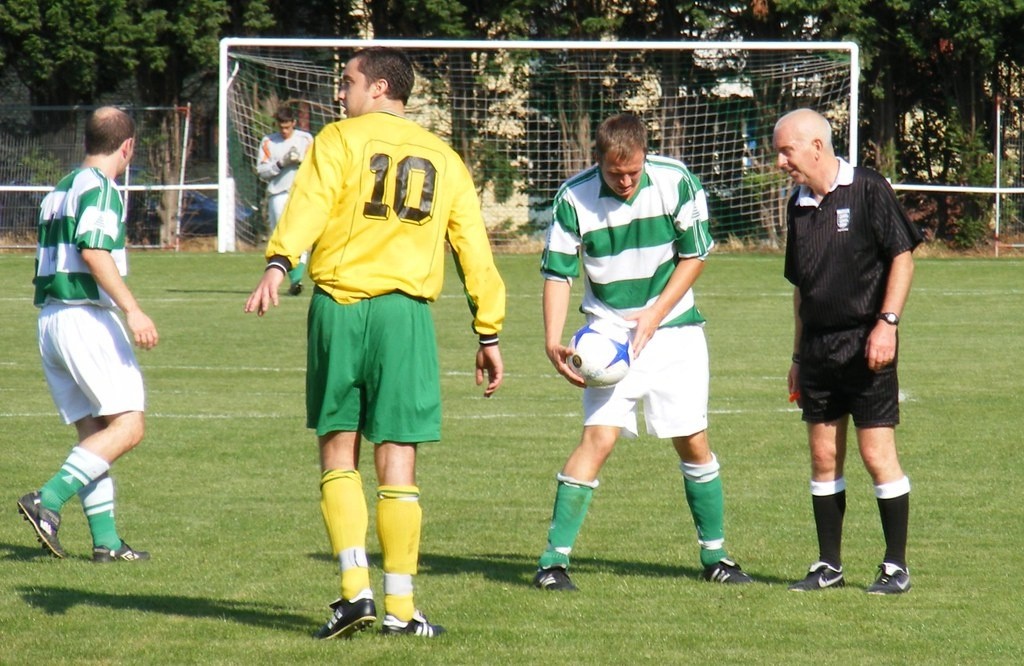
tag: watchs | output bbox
[878,312,899,325]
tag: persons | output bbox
[15,106,159,563]
[245,46,506,637]
[257,106,314,296]
[772,107,924,596]
[533,111,753,590]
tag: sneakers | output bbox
[18,490,68,560]
[381,604,447,638]
[789,561,845,593]
[315,595,377,639]
[867,562,912,595]
[704,554,752,583]
[93,537,150,564]
[532,562,580,592]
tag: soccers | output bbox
[566,321,632,387]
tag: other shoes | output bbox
[290,282,303,296]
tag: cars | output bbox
[118,167,254,245]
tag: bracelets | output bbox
[793,353,800,363]
